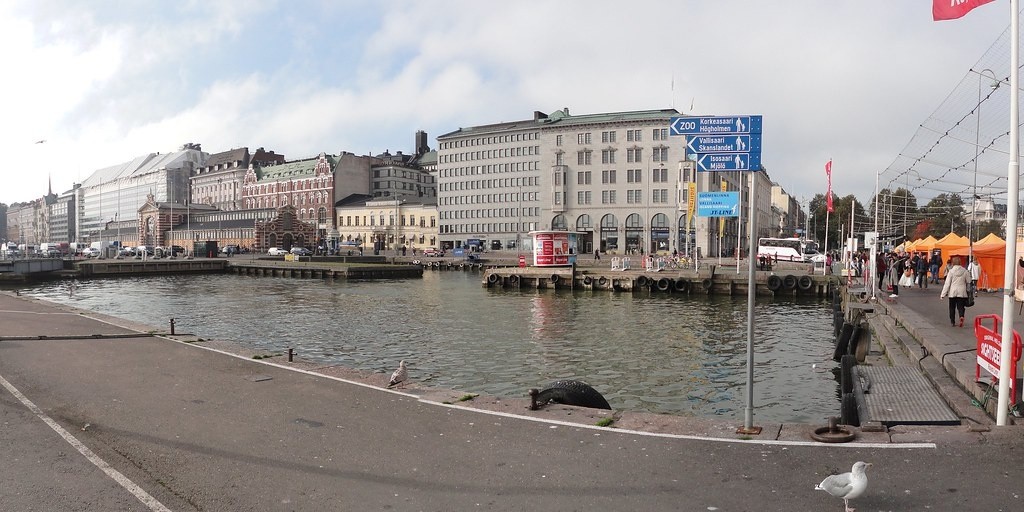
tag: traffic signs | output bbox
[669,115,762,172]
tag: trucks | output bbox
[56,242,69,252]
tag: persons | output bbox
[318,244,363,256]
[760,251,778,273]
[940,257,972,327]
[236,244,240,254]
[724,247,735,257]
[594,248,599,260]
[672,248,678,256]
[401,245,415,255]
[628,247,644,255]
[827,249,981,297]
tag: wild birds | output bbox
[386,360,410,389]
[815,461,873,512]
[35,140,46,143]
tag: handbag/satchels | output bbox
[963,289,974,307]
[915,275,924,284]
[905,272,910,277]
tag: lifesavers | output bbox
[551,274,559,282]
[599,277,606,285]
[413,259,484,268]
[703,278,712,289]
[766,274,813,291]
[583,277,591,285]
[637,275,648,287]
[840,354,858,398]
[536,380,612,410]
[675,279,688,292]
[657,277,670,291]
[489,273,498,283]
[830,289,844,337]
[840,392,859,427]
[510,275,517,283]
[833,322,854,361]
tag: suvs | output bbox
[223,245,245,254]
[169,245,184,255]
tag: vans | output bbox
[423,247,444,257]
[91,241,109,255]
[7,240,56,253]
[69,243,87,252]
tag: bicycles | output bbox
[664,257,701,270]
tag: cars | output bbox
[268,247,288,256]
[119,245,152,255]
[290,247,313,256]
[82,248,100,257]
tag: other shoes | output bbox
[889,294,898,298]
[951,320,955,326]
[960,316,964,326]
[975,291,977,297]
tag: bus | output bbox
[758,238,819,262]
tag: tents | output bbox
[896,232,1006,292]
